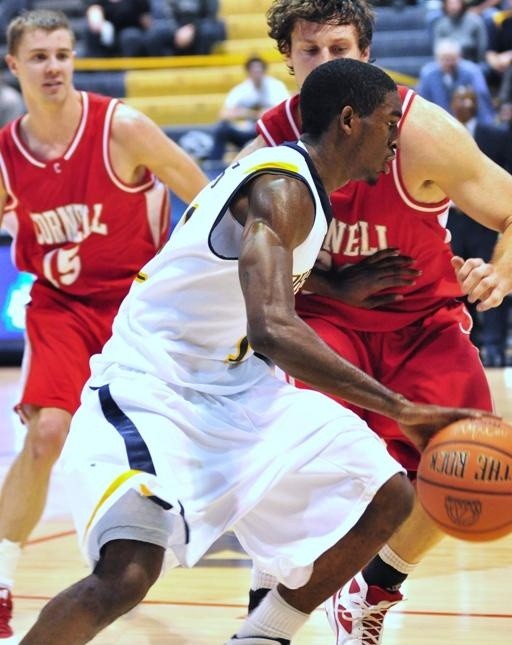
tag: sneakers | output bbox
[0,584,13,640]
[325,572,405,645]
[227,633,291,645]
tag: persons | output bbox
[13,59,502,645]
[0,1,512,173]
[227,0,512,645]
[0,10,219,638]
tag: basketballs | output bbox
[416,417,511,541]
